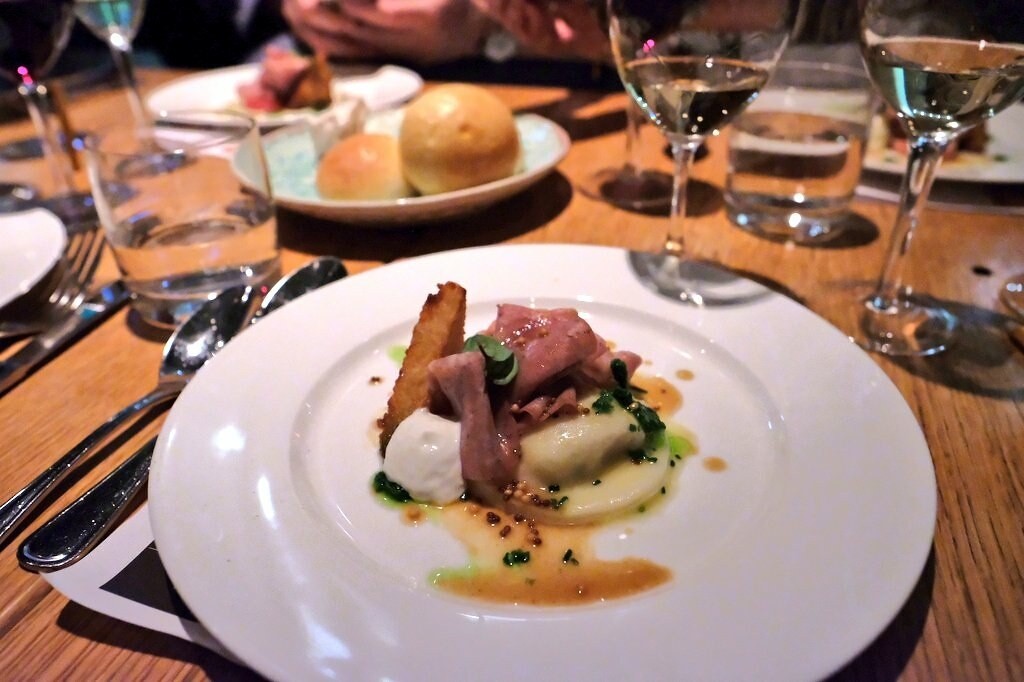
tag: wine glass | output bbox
[576,0,692,211]
[804,0,1024,358]
[604,0,804,256]
[0,0,201,214]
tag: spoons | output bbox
[0,284,263,548]
[15,258,348,572]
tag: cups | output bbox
[73,111,280,331]
[721,59,876,243]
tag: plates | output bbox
[144,62,424,129]
[0,204,67,309]
[230,107,571,223]
[146,243,939,682]
[748,87,1024,184]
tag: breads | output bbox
[378,281,465,454]
[312,133,423,200]
[398,78,519,195]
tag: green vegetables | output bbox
[369,334,667,565]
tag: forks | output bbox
[0,224,106,338]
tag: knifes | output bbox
[0,280,131,395]
